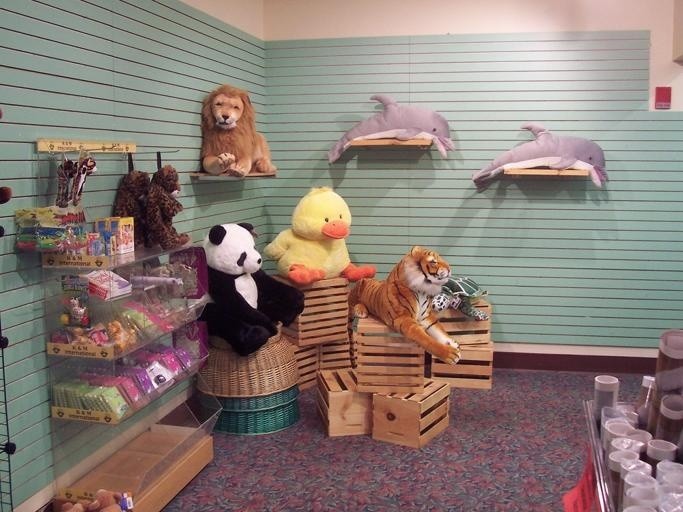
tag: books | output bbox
[40,215,194,420]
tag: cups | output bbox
[590,328,683,511]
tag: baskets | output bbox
[190,321,302,436]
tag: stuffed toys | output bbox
[51,488,133,511]
[110,170,150,252]
[346,244,461,366]
[144,164,191,251]
[200,85,277,177]
[52,147,96,209]
[261,185,375,287]
[473,124,609,189]
[198,221,304,358]
[323,94,455,166]
[428,273,488,323]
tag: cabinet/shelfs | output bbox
[41,242,225,512]
